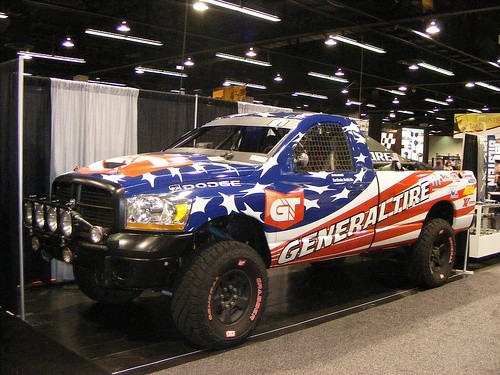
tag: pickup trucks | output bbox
[23,113,478,352]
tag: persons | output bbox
[443,161,453,171]
[435,160,444,170]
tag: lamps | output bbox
[215,52,273,68]
[17,50,85,63]
[225,79,267,89]
[329,33,385,54]
[201,0,281,22]
[135,66,188,77]
[85,28,163,46]
[417,62,454,76]
[308,72,350,83]
